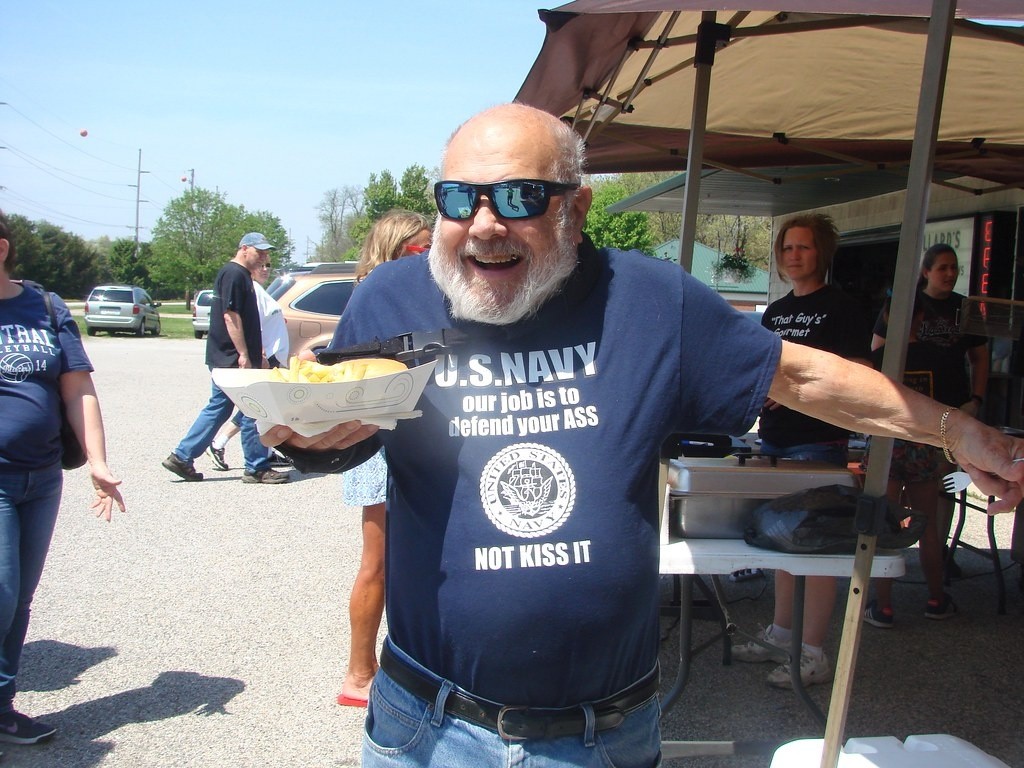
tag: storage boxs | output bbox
[209,358,442,437]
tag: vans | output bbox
[83,285,162,337]
[191,289,216,338]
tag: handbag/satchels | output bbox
[742,484,928,554]
[44,292,87,470]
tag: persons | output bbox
[466,185,474,206]
[258,102,1024,768]
[870,244,989,578]
[506,185,515,205]
[862,292,958,628]
[162,232,290,484]
[731,213,874,689]
[0,208,126,745]
[204,253,293,471]
[337,210,434,707]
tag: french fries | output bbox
[269,355,366,384]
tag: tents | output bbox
[511,0,1024,768]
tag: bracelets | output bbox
[974,394,986,406]
[939,406,958,466]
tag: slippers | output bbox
[337,694,368,708]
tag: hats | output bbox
[239,232,276,251]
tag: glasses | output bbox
[434,178,580,221]
[262,263,270,267]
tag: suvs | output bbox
[266,260,368,369]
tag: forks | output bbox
[942,457,1024,494]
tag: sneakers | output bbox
[265,452,290,466]
[205,441,228,471]
[766,648,832,690]
[162,452,203,481]
[242,468,289,484]
[0,710,57,744]
[924,598,959,619]
[731,622,792,665]
[863,602,894,628]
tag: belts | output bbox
[380,638,659,738]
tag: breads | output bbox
[330,357,408,380]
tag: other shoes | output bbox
[943,544,961,581]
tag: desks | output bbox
[943,461,1024,615]
[658,487,907,742]
